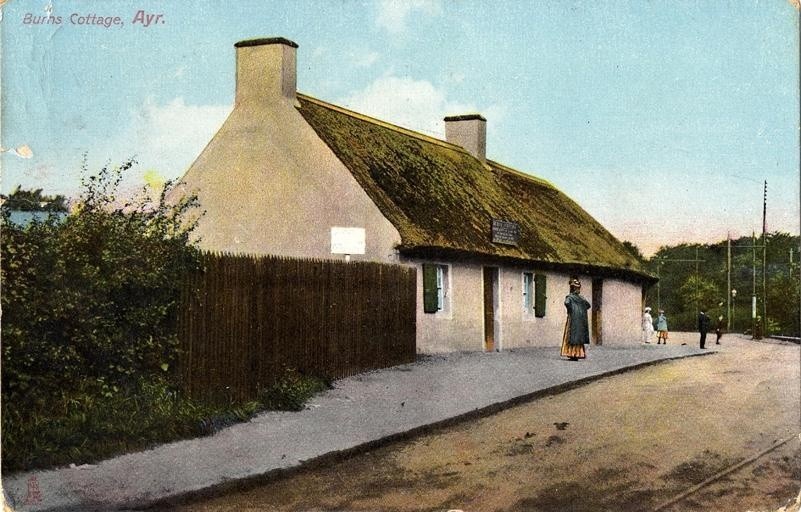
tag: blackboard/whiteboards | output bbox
[491,220,518,247]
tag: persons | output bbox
[716,315,724,344]
[657,309,669,344]
[755,315,763,341]
[643,306,655,343]
[699,308,710,349]
[562,279,592,362]
